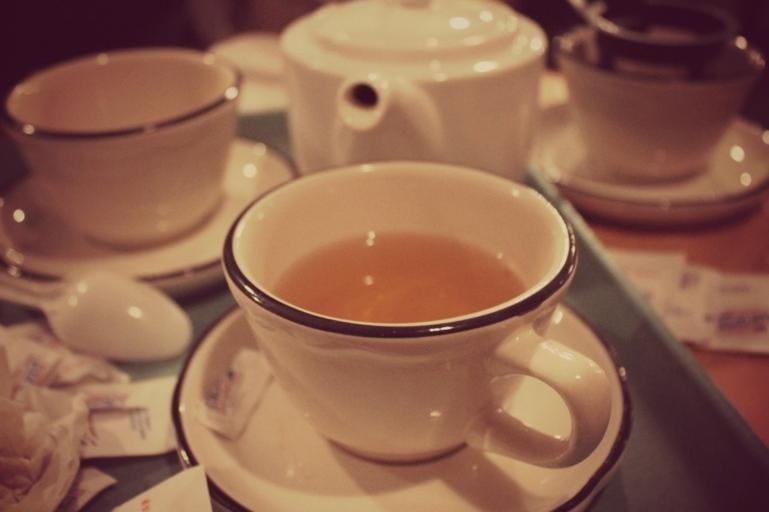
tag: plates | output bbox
[172,286,635,512]
[0,138,302,293]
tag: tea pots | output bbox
[282,0,548,187]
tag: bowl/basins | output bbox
[2,49,244,228]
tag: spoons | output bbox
[0,273,194,365]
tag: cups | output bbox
[536,109,764,229]
[556,13,765,185]
[225,159,611,466]
